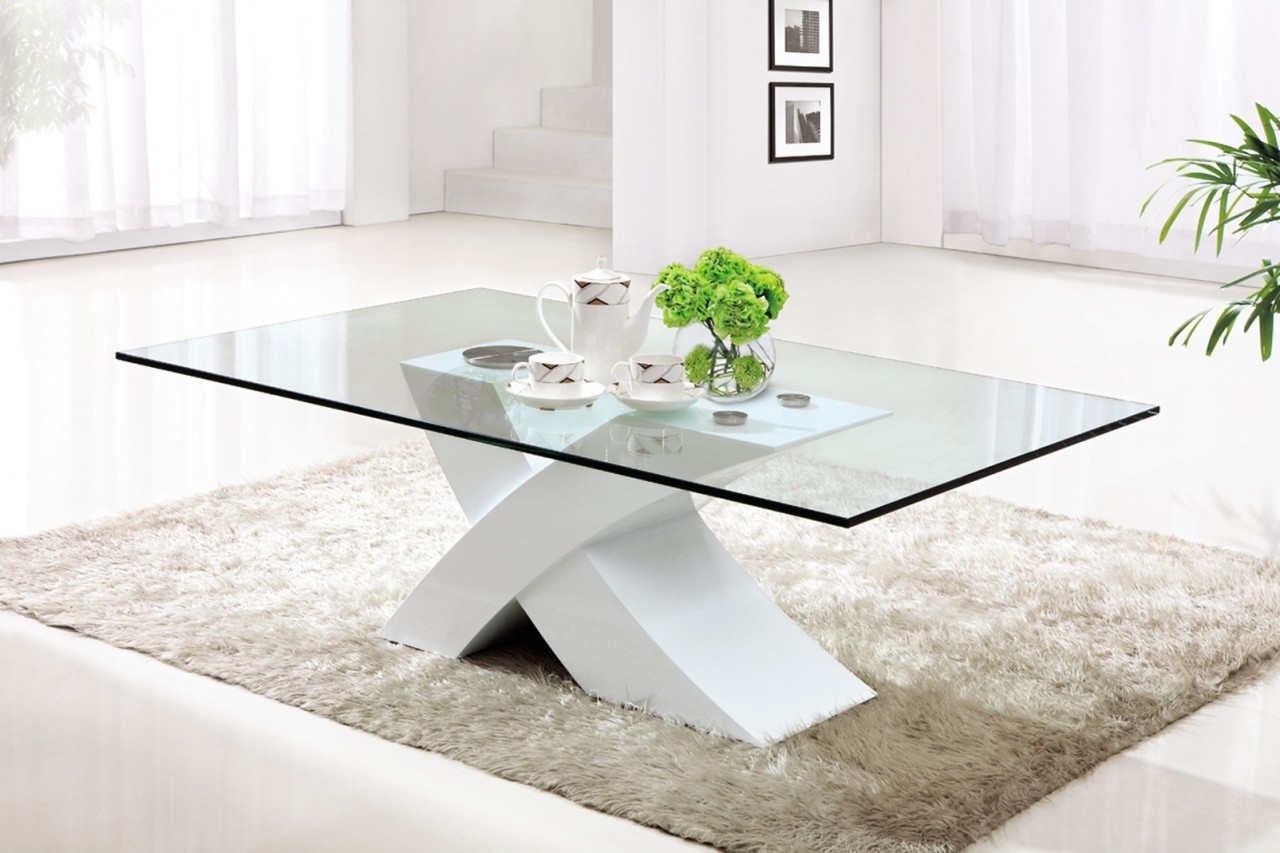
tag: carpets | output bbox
[0,436,1278,853]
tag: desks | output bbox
[111,291,1159,753]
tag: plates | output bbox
[506,379,606,409]
[609,380,706,411]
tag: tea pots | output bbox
[536,255,672,380]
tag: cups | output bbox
[511,352,586,397]
[611,353,685,398]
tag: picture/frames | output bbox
[765,0,835,73]
[768,81,835,163]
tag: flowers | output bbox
[651,248,783,402]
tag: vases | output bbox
[669,323,774,399]
